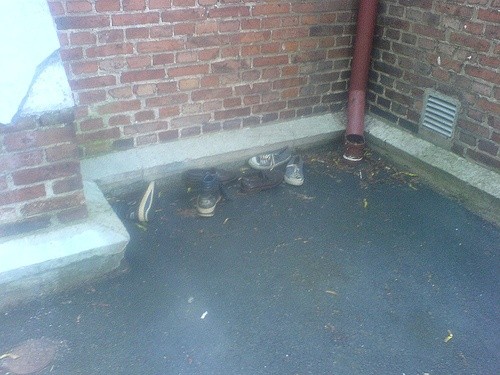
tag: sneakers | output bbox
[197,169,223,216]
[286,153,306,186]
[249,146,291,172]
[127,182,157,224]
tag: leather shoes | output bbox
[239,173,283,194]
[184,168,236,187]
